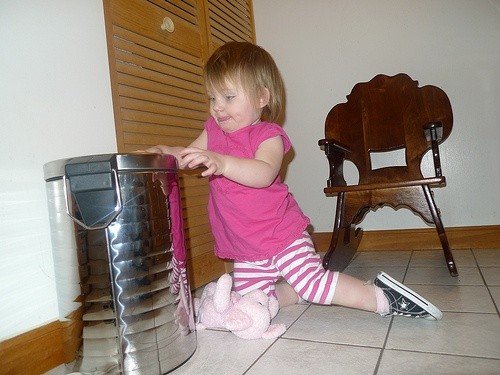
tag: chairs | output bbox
[322,73,458,278]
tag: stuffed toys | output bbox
[191,271,287,341]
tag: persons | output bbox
[132,40,443,320]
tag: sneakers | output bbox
[373,270,442,321]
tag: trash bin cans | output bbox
[42,152,199,375]
